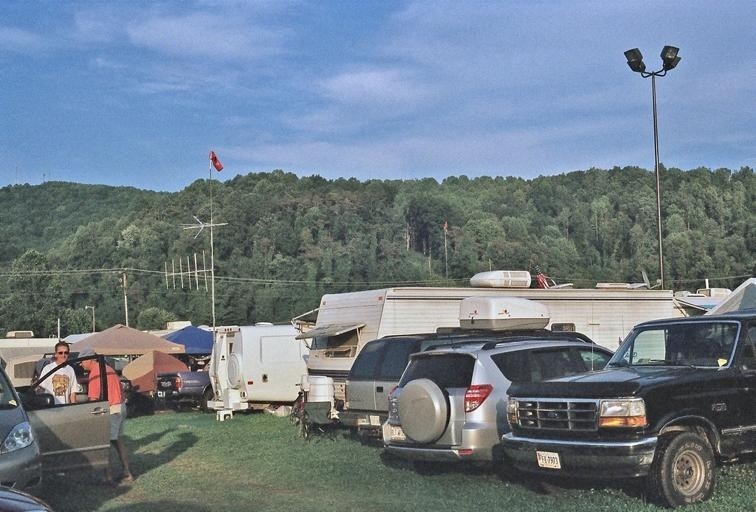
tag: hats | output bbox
[75,349,95,364]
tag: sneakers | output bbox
[95,473,133,486]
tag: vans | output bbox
[339,331,596,442]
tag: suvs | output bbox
[501,312,756,511]
[382,330,629,470]
[30,351,141,416]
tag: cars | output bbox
[0,353,112,490]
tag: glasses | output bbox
[58,351,69,354]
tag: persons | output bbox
[35,342,82,476]
[77,349,134,484]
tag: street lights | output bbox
[85,305,96,332]
[623,44,681,293]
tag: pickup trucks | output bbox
[155,371,214,413]
